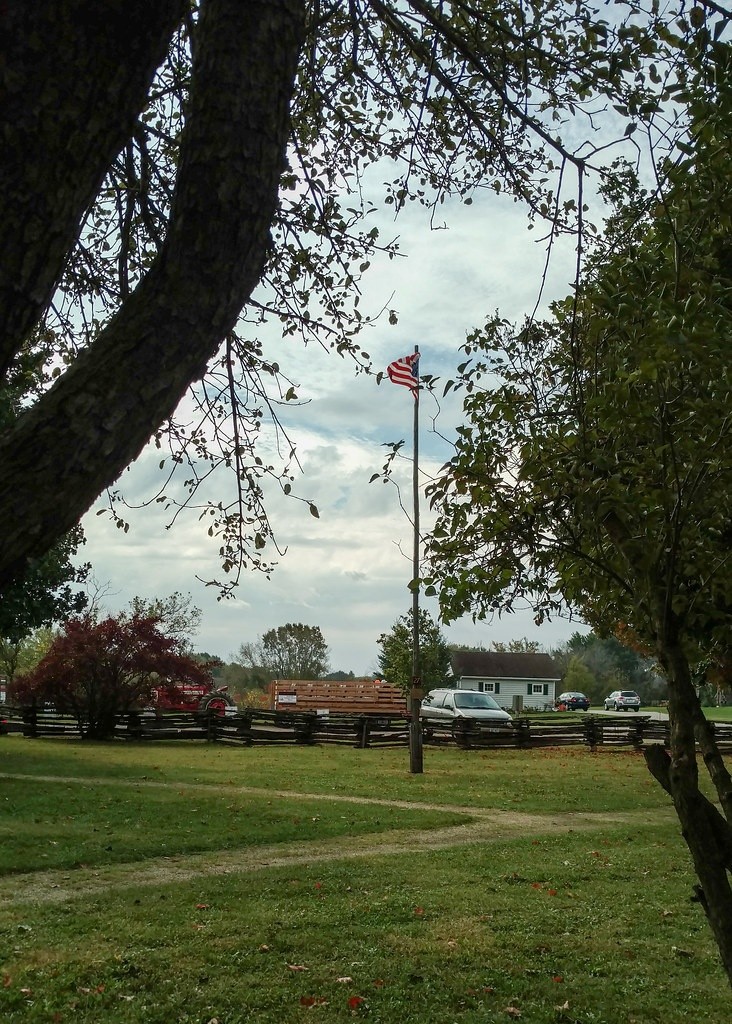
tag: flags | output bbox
[387,352,421,400]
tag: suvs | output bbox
[604,690,641,712]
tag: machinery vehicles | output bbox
[139,680,237,726]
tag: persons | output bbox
[374,680,391,703]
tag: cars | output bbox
[556,692,590,711]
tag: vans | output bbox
[419,688,515,743]
